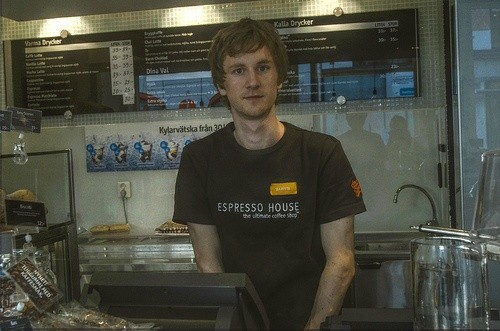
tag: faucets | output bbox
[393,185,439,226]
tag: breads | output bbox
[90,225,110,231]
[110,224,130,231]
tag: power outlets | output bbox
[118,181,131,198]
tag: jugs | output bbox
[470,147,500,262]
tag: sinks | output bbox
[349,239,411,255]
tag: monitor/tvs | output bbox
[79,270,272,331]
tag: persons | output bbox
[333,112,385,183]
[385,115,424,174]
[171,17,367,331]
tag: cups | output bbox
[411,236,490,331]
